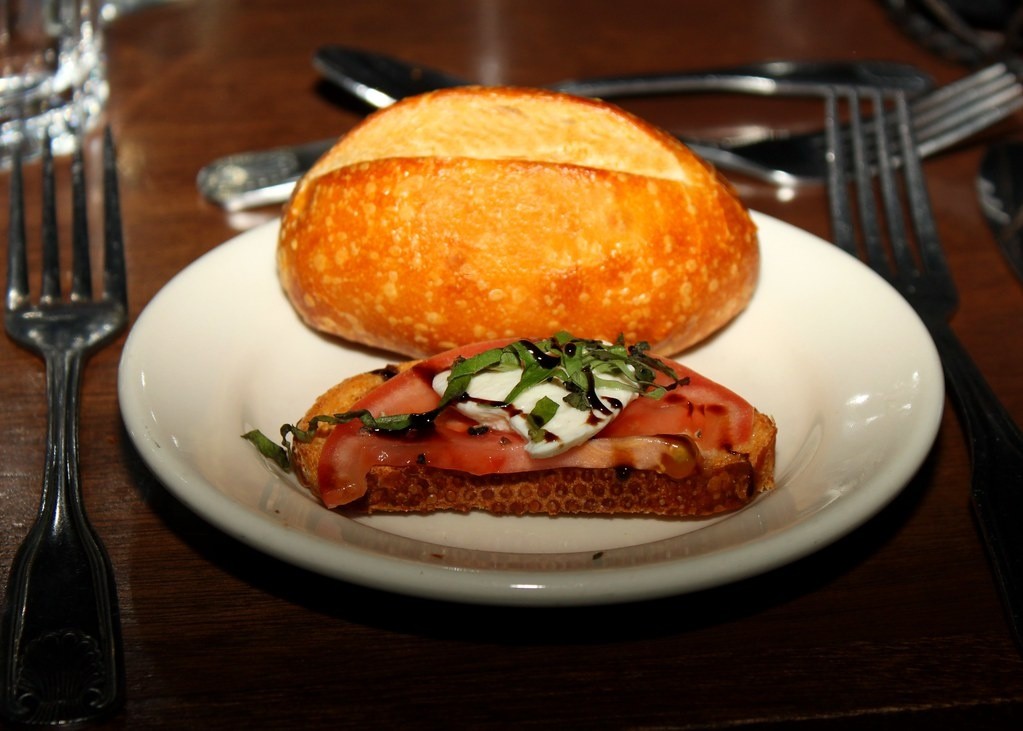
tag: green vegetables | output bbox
[243,330,690,476]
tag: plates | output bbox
[115,205,946,607]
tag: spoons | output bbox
[309,43,935,109]
[976,139,1023,285]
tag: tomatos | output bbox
[317,333,755,508]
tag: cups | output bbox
[0,0,104,173]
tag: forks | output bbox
[822,83,1023,661]
[194,60,1023,214]
[4,126,130,726]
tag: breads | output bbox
[279,84,761,361]
[294,361,777,518]
